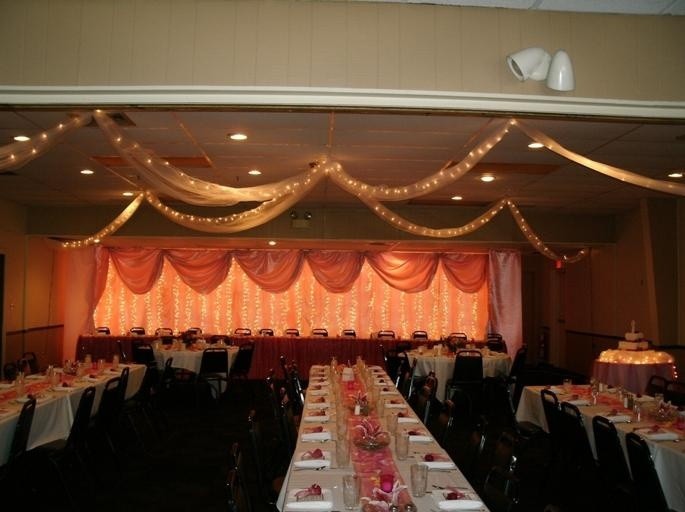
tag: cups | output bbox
[14,353,120,401]
[416,344,488,359]
[560,376,662,423]
[326,354,429,510]
[151,336,225,352]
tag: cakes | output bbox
[618,331,649,349]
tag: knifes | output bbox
[427,467,456,473]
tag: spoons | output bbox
[432,482,468,490]
[292,404,327,473]
[655,439,680,443]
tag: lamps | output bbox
[505,48,574,92]
[288,209,297,220]
[304,209,313,219]
